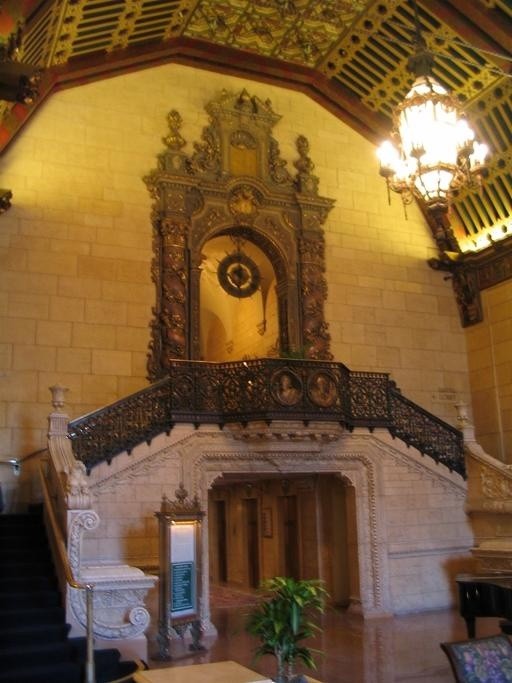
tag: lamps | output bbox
[374,9,490,244]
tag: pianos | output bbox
[455,571,510,638]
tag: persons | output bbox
[311,375,335,405]
[278,373,300,402]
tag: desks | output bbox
[131,660,275,683]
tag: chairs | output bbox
[437,631,512,682]
